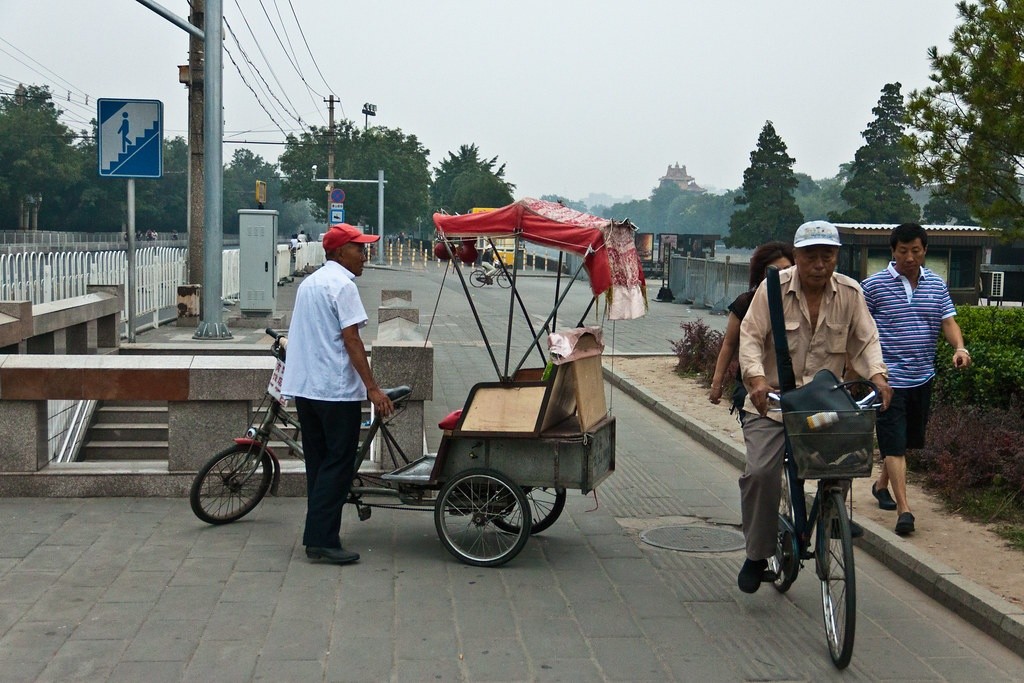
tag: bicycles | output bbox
[758,389,884,668]
[469,262,513,289]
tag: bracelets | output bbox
[711,384,720,389]
[957,349,969,355]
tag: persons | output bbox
[481,245,497,277]
[859,223,972,532]
[738,219,894,593]
[281,223,394,562]
[706,241,795,429]
[172,229,178,240]
[398,232,404,244]
[124,229,158,241]
[289,231,312,255]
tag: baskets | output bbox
[784,409,876,479]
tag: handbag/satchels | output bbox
[783,370,879,463]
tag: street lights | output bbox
[361,102,377,132]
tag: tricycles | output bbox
[186,197,648,567]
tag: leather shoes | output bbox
[873,481,898,511]
[305,545,359,563]
[896,513,915,534]
[831,518,864,539]
[737,558,769,594]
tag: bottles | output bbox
[807,412,839,431]
[807,448,825,464]
[835,448,867,467]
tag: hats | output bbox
[322,224,380,253]
[794,221,840,247]
